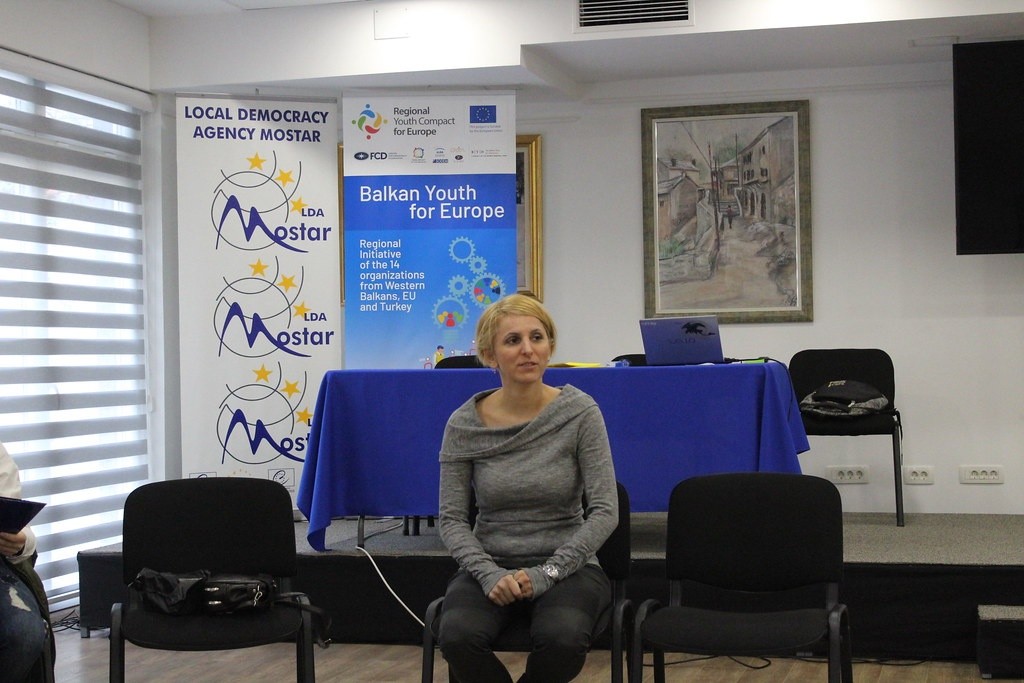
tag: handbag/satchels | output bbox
[203,572,276,612]
[799,380,889,416]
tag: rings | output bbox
[17,544,21,550]
[518,582,523,589]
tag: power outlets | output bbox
[824,465,869,484]
[959,464,1004,484]
[903,466,934,484]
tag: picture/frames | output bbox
[337,133,542,305]
[641,100,813,324]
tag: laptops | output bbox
[639,315,746,366]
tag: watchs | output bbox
[540,563,559,584]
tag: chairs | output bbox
[788,348,905,527]
[629,472,854,683]
[421,481,636,683]
[109,478,315,683]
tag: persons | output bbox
[439,295,619,683]
[0,442,45,683]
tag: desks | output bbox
[297,362,810,552]
